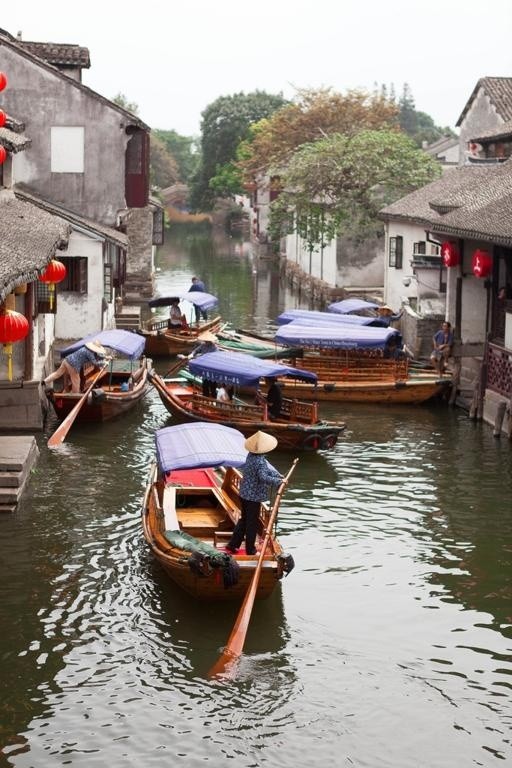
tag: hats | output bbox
[84,340,106,354]
[244,430,278,454]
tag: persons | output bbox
[170,277,208,329]
[430,321,454,377]
[188,341,234,410]
[378,305,405,327]
[264,377,282,419]
[225,431,289,556]
[41,341,113,393]
[385,341,406,360]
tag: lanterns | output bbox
[0,309,30,344]
[0,71,7,163]
[38,260,67,284]
[440,240,461,267]
[471,247,493,278]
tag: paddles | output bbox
[47,359,112,446]
[225,456,299,655]
